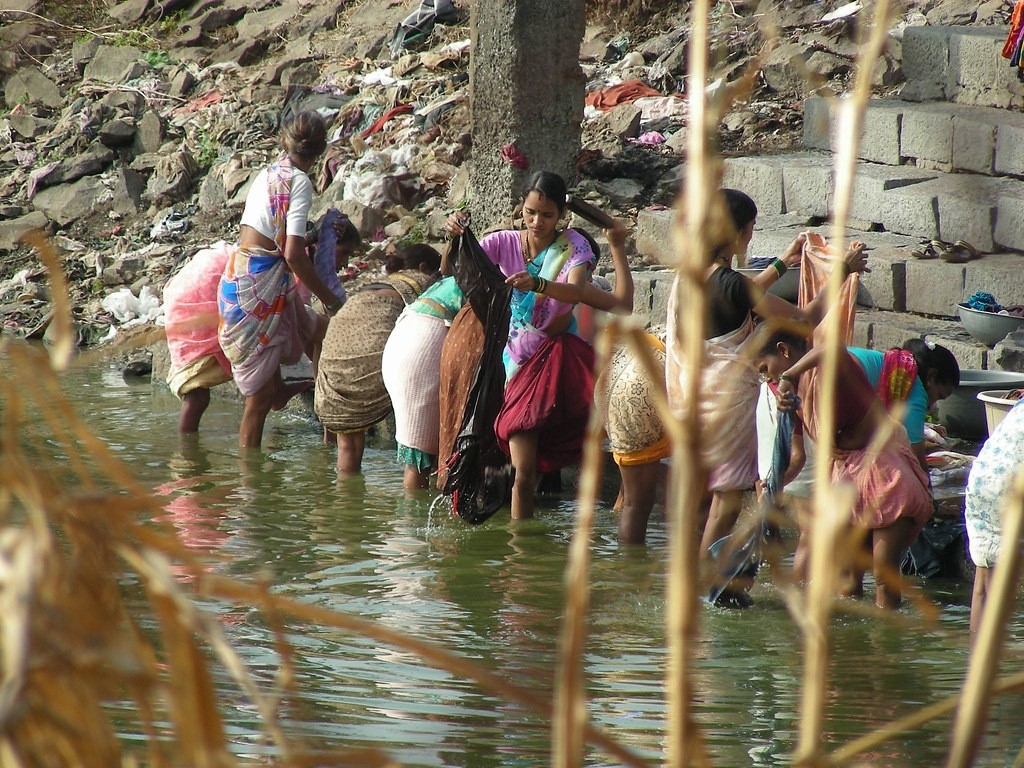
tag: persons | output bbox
[965,394,1024,636]
[162,112,956,614]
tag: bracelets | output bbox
[842,261,850,279]
[779,375,794,386]
[324,295,341,311]
[770,258,787,279]
[531,276,546,293]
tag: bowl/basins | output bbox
[956,302,1024,346]
[976,390,1019,438]
[734,266,800,302]
[936,369,1024,440]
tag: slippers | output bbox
[911,239,982,262]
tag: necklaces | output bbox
[524,225,558,277]
